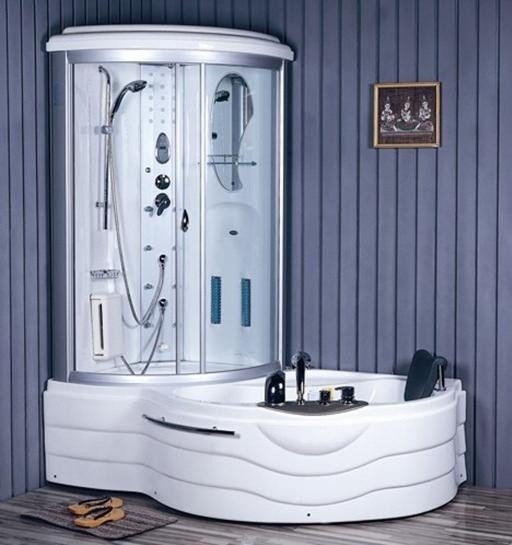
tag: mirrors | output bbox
[210,74,254,192]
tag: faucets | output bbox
[291,352,311,405]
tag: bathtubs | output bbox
[42,369,468,525]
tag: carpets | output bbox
[19,501,179,540]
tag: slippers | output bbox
[73,506,125,529]
[67,497,123,516]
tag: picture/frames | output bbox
[373,82,440,149]
